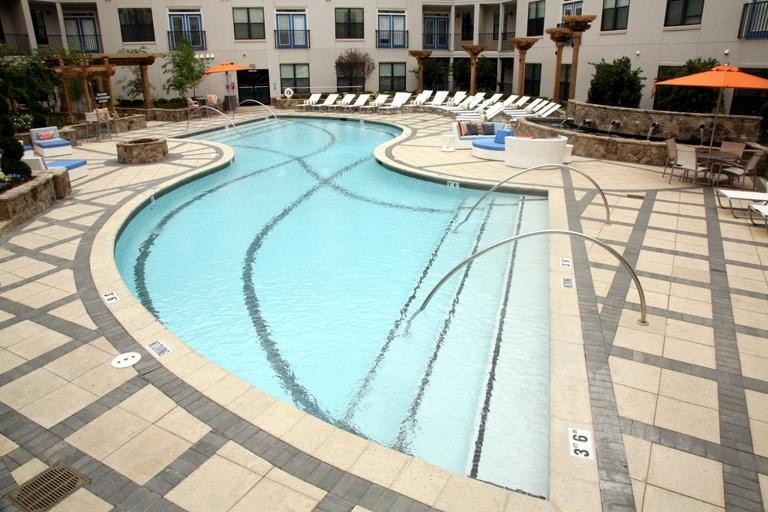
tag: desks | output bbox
[685,148,739,186]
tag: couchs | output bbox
[450,121,508,149]
[503,135,568,171]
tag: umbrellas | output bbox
[205,60,256,111]
[653,63,768,178]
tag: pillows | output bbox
[37,131,55,140]
[457,121,496,136]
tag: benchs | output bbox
[471,128,515,162]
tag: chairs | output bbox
[721,149,764,190]
[294,91,561,126]
[29,125,73,158]
[23,146,35,157]
[668,143,715,189]
[715,141,746,183]
[662,137,690,181]
[83,107,118,143]
[208,99,220,116]
[186,97,201,120]
[747,204,768,227]
[715,188,768,218]
[21,156,87,172]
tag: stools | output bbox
[441,134,455,151]
[563,144,574,164]
